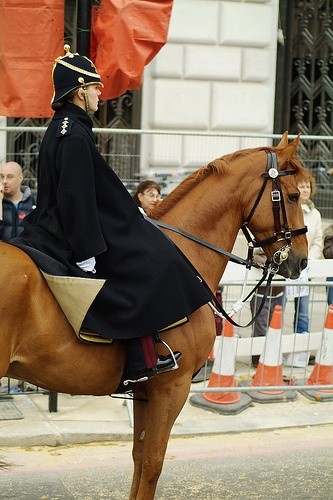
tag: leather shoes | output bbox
[132,351,183,378]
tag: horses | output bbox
[0,130,314,500]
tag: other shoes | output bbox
[18,384,35,391]
[308,355,316,361]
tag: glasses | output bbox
[142,192,162,199]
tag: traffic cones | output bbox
[295,304,333,401]
[238,305,297,403]
[190,318,252,414]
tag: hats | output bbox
[50,44,105,113]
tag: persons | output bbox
[0,162,36,391]
[250,167,333,368]
[22,44,212,380]
[133,181,161,213]
